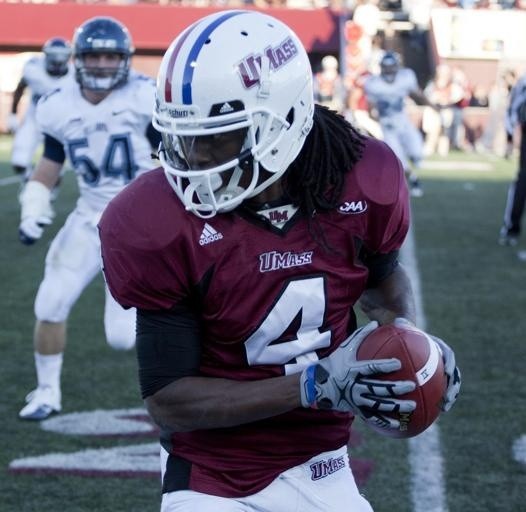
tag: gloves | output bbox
[396,316,465,414]
[298,319,417,430]
[17,213,53,245]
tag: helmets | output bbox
[379,54,399,84]
[70,14,138,94]
[41,36,70,77]
[149,7,319,222]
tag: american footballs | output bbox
[358,323,447,440]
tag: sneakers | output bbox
[411,187,424,198]
[17,389,63,422]
